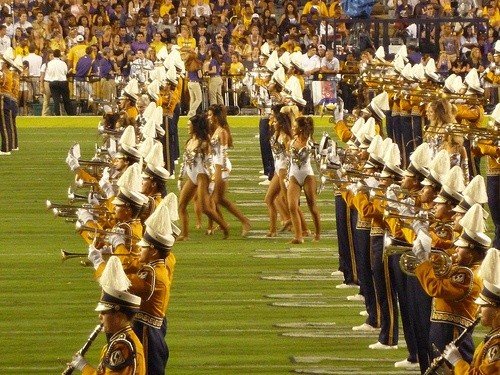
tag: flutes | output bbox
[422,317,480,375]
[60,322,103,375]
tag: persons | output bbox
[1,0,500,374]
[66,255,147,375]
[442,247,500,374]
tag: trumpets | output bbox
[305,141,460,278]
[45,69,141,267]
[341,72,500,168]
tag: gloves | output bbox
[362,71,419,101]
[444,342,463,365]
[66,105,126,271]
[331,152,429,264]
[66,352,87,370]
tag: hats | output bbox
[475,248,500,307]
[259,42,308,110]
[348,117,488,213]
[111,45,185,249]
[94,255,141,311]
[374,38,500,95]
[453,202,492,249]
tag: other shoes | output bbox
[331,270,344,276]
[359,309,369,317]
[394,359,420,368]
[352,321,382,331]
[347,293,364,301]
[368,341,398,349]
[336,283,359,289]
